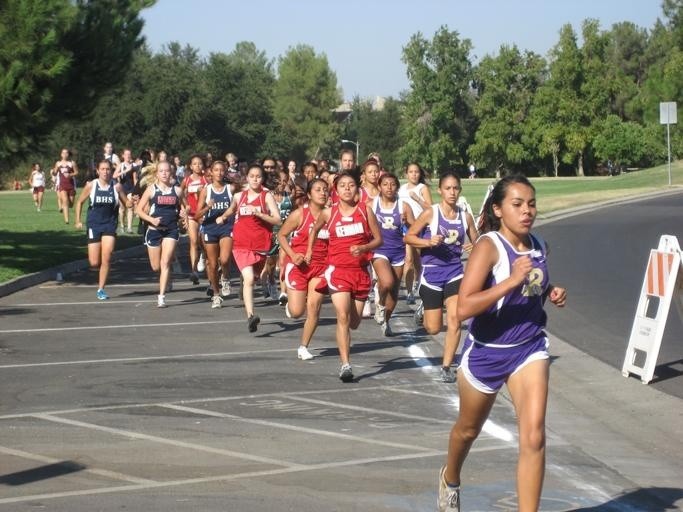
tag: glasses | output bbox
[263,165,276,170]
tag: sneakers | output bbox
[439,365,455,383]
[120,225,133,234]
[436,463,462,512]
[278,293,288,307]
[212,273,232,308]
[373,302,393,337]
[361,299,373,319]
[338,363,353,380]
[191,272,214,298]
[414,300,424,327]
[156,294,167,308]
[406,281,420,305]
[297,346,313,360]
[197,252,206,273]
[96,288,108,300]
[248,314,261,332]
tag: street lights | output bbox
[340,139,359,165]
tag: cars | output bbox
[590,159,623,178]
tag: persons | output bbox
[436,175,568,512]
[76,142,294,333]
[277,146,479,383]
[28,148,78,223]
